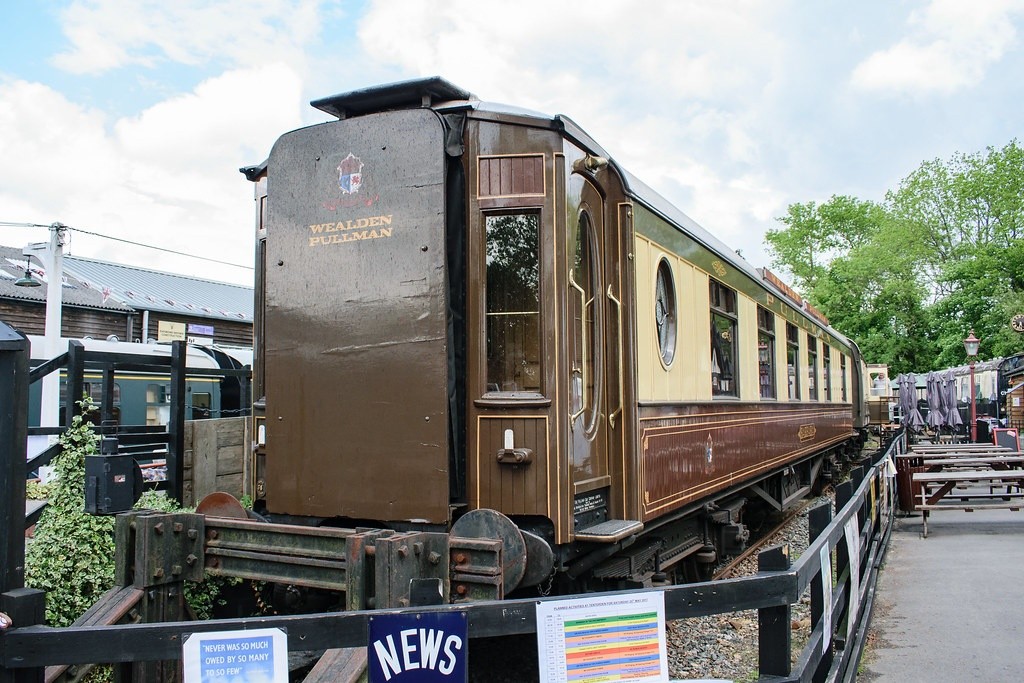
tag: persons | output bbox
[874,373,893,400]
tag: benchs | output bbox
[915,502,1024,510]
[59,405,119,425]
[926,482,1019,488]
[915,493,1024,499]
[925,467,993,471]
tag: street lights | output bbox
[15,222,66,487]
[962,330,982,444]
[196,77,868,610]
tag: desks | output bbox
[908,443,1024,539]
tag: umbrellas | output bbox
[896,370,963,444]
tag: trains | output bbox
[25,334,253,537]
